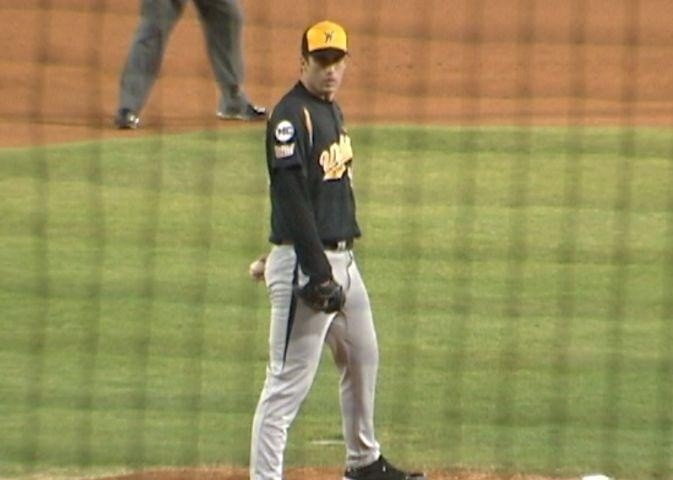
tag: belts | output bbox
[320,238,354,251]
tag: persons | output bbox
[247,20,426,479]
[113,0,269,130]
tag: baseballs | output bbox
[250,260,264,280]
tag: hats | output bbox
[302,21,348,57]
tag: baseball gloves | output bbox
[298,282,345,313]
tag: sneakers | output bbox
[216,104,269,121]
[342,455,427,480]
[114,108,140,128]
